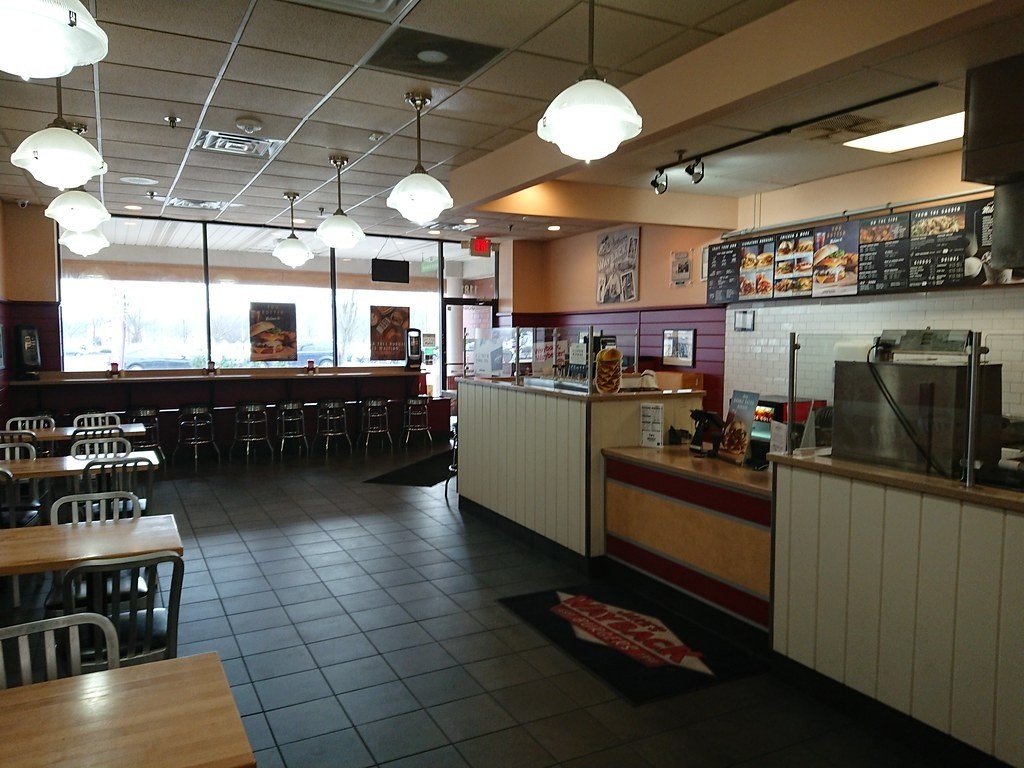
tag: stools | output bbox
[276,402,309,454]
[314,398,352,450]
[444,423,458,498]
[230,401,275,459]
[125,407,166,460]
[172,402,221,462]
[357,396,393,446]
[401,392,433,444]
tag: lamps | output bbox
[0,2,109,81]
[274,191,315,268]
[386,89,454,230]
[11,77,109,193]
[531,1,645,162]
[54,225,112,257]
[43,185,112,231]
[312,154,369,253]
[651,171,668,196]
[684,159,709,185]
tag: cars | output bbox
[124,357,193,370]
[263,340,339,368]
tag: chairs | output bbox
[72,412,122,428]
[60,550,185,679]
[69,428,125,447]
[73,439,131,496]
[1,444,38,498]
[6,415,55,430]
[80,459,156,511]
[43,490,150,622]
[0,611,123,692]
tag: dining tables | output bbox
[1,448,160,478]
[33,366,429,384]
[1,422,147,444]
[0,650,258,768]
[1,514,184,577]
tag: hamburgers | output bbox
[595,350,623,394]
[251,321,286,355]
[723,422,749,458]
[740,240,853,294]
